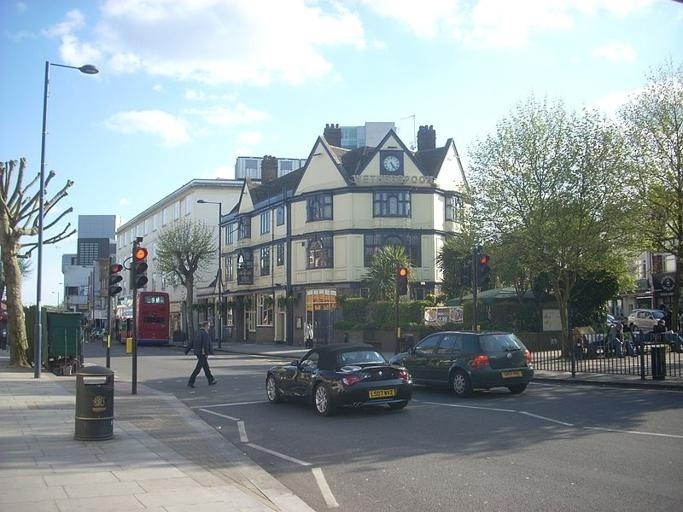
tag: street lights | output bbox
[33,58,99,381]
[50,291,60,310]
[57,281,68,311]
[195,199,224,348]
[78,263,95,324]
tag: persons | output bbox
[607,323,638,358]
[184,321,217,389]
[655,319,683,353]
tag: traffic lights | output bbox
[132,246,150,289]
[107,261,122,298]
[396,264,410,295]
[474,253,490,287]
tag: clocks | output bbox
[384,155,400,172]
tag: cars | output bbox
[597,312,627,333]
[387,330,535,398]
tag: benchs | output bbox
[631,330,670,355]
[583,333,609,360]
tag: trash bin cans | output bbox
[399,334,414,353]
[73,366,114,441]
[651,345,667,380]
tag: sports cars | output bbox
[263,342,412,415]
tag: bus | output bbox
[111,291,170,350]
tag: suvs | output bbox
[624,307,671,331]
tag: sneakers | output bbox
[187,379,218,388]
